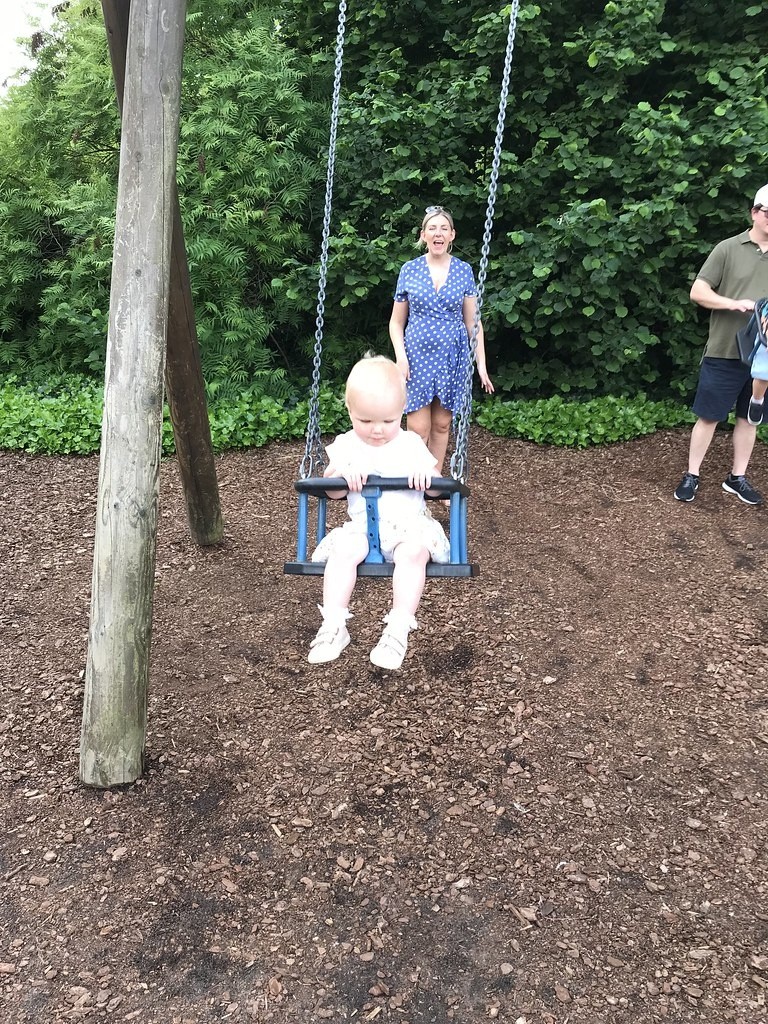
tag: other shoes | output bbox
[423,490,450,501]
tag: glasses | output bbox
[756,207,768,217]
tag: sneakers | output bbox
[721,474,764,505]
[307,617,351,663]
[747,398,766,425]
[674,471,699,501]
[370,625,411,671]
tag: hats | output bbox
[754,184,768,207]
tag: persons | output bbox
[309,350,451,671]
[674,183,768,506]
[747,302,768,425]
[388,203,495,507]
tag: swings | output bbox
[284,0,518,577]
[735,296,768,364]
